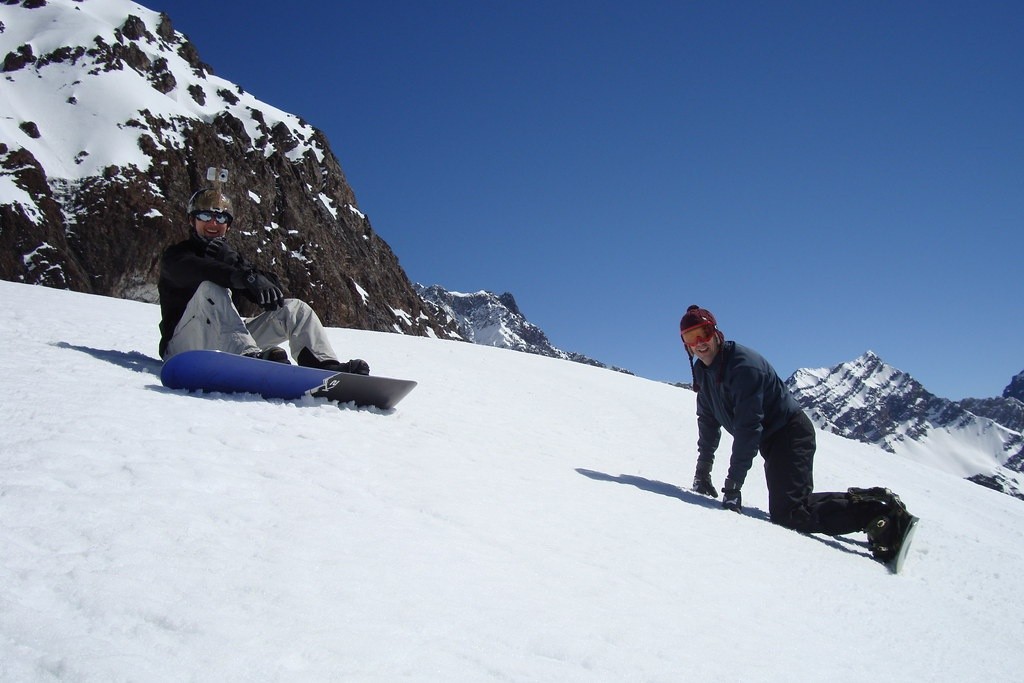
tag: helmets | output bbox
[186,187,234,227]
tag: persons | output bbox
[680,304,921,574]
[157,189,370,379]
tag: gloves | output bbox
[204,239,251,269]
[693,470,718,498]
[242,268,285,312]
[722,479,743,514]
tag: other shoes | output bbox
[245,345,291,364]
[848,486,907,561]
[296,346,370,375]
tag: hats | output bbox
[680,304,725,392]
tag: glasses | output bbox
[681,321,716,346]
[194,211,229,224]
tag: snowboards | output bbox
[881,486,921,575]
[160,349,417,409]
